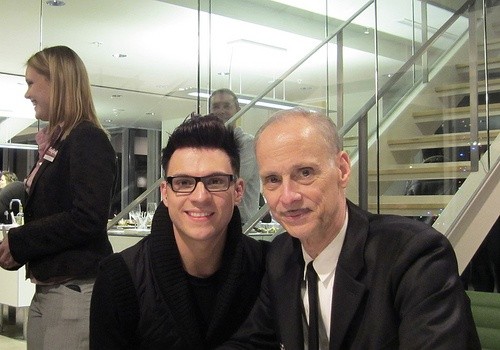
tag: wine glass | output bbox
[130,203,141,230]
[147,202,157,230]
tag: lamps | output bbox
[178,39,337,116]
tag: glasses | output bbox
[166,174,235,193]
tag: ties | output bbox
[306,262,321,349]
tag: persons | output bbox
[90,111,287,350]
[0,169,19,193]
[0,44,121,350]
[208,89,260,234]
[214,109,483,350]
[0,176,29,225]
